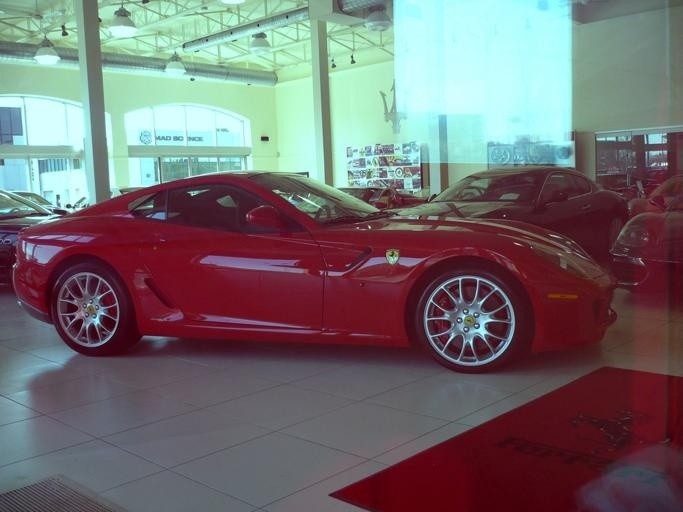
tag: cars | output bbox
[595,165,668,200]
[609,195,682,296]
[66,185,154,211]
[1,191,64,275]
[397,165,627,255]
[628,176,681,215]
[341,187,428,208]
[11,192,70,218]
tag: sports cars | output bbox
[8,163,622,374]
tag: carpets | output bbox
[326,366,682,512]
